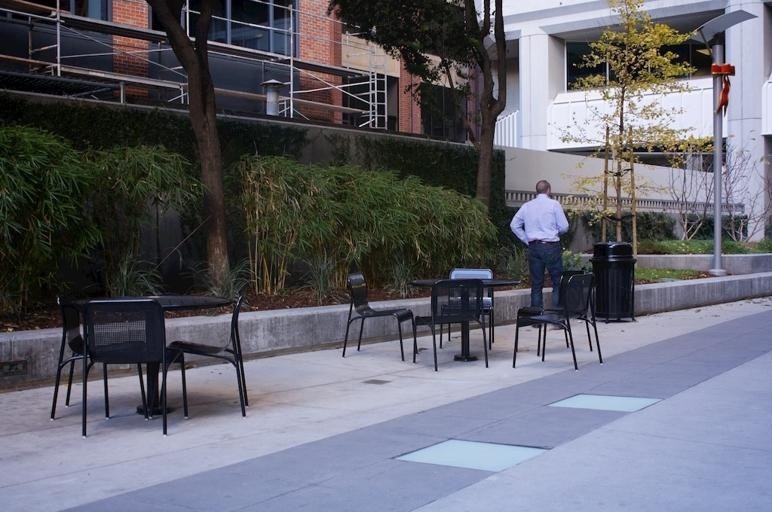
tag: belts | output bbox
[528,240,558,246]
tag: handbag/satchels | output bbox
[517,304,544,316]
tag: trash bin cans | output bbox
[589,242,637,323]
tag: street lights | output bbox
[688,9,758,278]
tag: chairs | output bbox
[51,295,248,437]
[342,269,603,372]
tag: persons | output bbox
[510,180,570,329]
